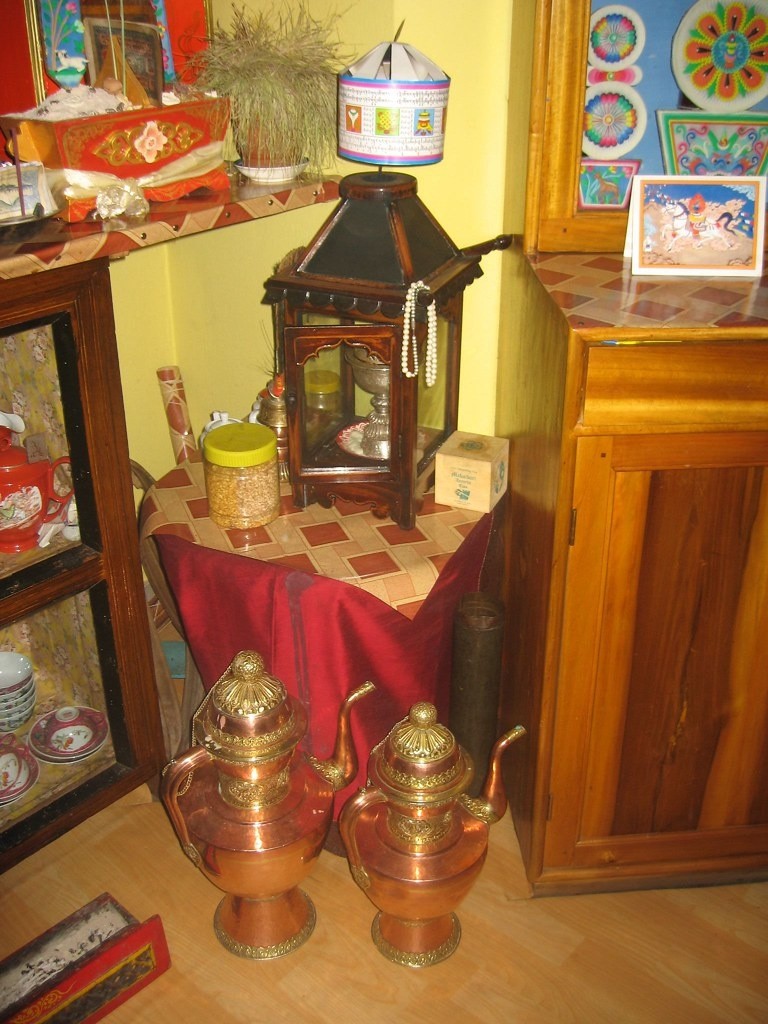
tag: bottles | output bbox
[201,423,281,528]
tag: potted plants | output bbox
[177,2,356,184]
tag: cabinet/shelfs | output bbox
[0,257,181,872]
[0,175,342,511]
[494,235,768,896]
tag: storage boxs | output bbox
[2,90,233,224]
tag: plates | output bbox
[336,419,431,464]
[1,705,110,808]
[233,155,309,184]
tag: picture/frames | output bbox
[656,110,766,174]
[25,0,212,112]
[523,0,767,258]
[632,173,763,279]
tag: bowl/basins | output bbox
[1,652,36,734]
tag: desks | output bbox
[136,411,510,854]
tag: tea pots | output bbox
[160,647,378,960]
[0,410,76,554]
[336,701,528,966]
[197,410,245,448]
